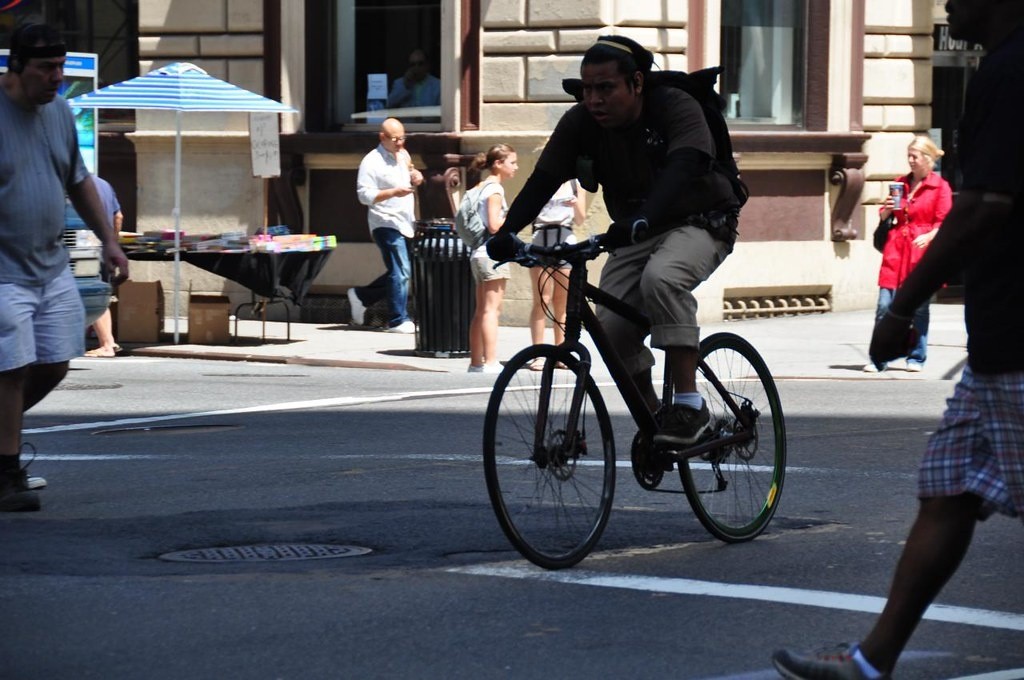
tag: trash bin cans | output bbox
[407,219,477,357]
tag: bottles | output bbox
[408,164,415,186]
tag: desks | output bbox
[124,247,335,342]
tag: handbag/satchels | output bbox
[874,212,895,250]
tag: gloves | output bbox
[605,217,648,245]
[487,234,525,260]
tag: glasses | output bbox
[408,60,426,67]
[384,133,406,142]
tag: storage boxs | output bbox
[188,280,231,343]
[110,281,164,343]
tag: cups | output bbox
[888,182,905,210]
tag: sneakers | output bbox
[771,637,892,680]
[22,473,48,489]
[653,397,711,444]
[383,320,415,334]
[468,365,485,373]
[348,288,367,324]
[482,362,504,373]
[0,470,41,512]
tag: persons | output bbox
[864,136,953,373]
[388,49,441,123]
[348,118,424,333]
[487,35,734,442]
[0,24,129,512]
[770,0,1024,680]
[529,180,587,371]
[457,143,518,374]
[84,174,123,357]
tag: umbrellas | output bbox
[67,62,298,344]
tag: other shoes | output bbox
[554,362,568,369]
[907,360,924,372]
[530,357,547,370]
[864,363,887,372]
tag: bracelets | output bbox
[886,308,911,320]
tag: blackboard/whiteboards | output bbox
[247,112,281,178]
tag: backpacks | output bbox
[454,182,497,248]
[562,67,750,207]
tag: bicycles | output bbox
[483,231,789,571]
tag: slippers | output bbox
[84,348,115,357]
[113,344,123,351]
